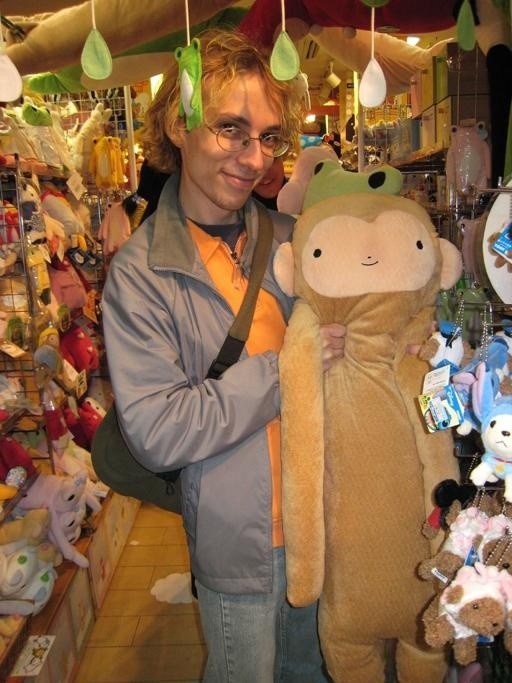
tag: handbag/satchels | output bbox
[91,395,185,515]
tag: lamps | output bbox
[319,62,341,99]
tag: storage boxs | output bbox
[409,42,490,148]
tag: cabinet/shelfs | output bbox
[0,79,144,683]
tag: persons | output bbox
[256,156,290,205]
[97,31,442,681]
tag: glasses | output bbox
[204,120,290,157]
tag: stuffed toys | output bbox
[0,0,512,130]
[0,88,138,617]
[0,89,140,618]
[336,101,396,169]
[271,164,465,683]
[446,118,494,208]
[277,143,338,215]
[421,313,512,664]
